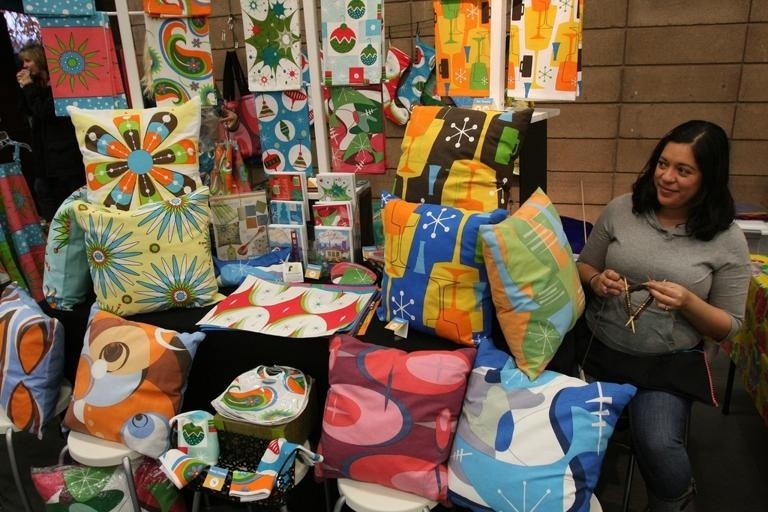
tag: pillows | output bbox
[66,94,202,210]
[446,337,637,512]
[43,185,90,311]
[380,187,509,347]
[73,186,225,317]
[479,187,586,381]
[31,459,189,512]
[1,280,65,439]
[391,104,535,213]
[315,333,476,506]
[62,303,206,459]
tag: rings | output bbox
[664,305,671,312]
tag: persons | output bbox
[16,43,90,209]
[574,119,752,512]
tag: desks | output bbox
[720,254,768,427]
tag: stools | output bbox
[333,476,442,512]
[0,377,72,512]
[602,342,710,512]
[58,429,147,512]
[191,440,312,512]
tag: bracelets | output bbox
[587,273,600,289]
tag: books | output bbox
[309,173,361,267]
[266,170,307,267]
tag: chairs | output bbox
[606,407,703,511]
